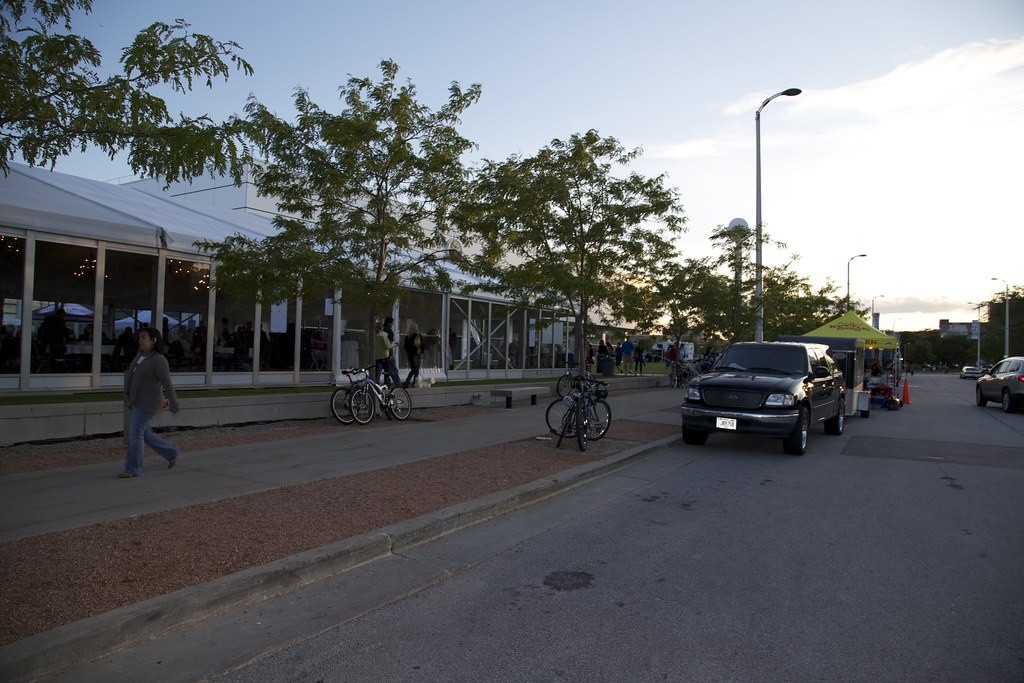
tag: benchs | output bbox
[490,387,550,408]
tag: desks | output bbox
[63,343,125,358]
[214,346,259,358]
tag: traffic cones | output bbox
[901,378,913,404]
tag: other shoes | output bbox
[168,456,176,469]
[412,383,414,388]
[119,472,135,478]
[406,381,409,384]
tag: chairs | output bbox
[310,347,328,370]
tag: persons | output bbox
[586,337,644,376]
[665,342,719,377]
[404,323,424,388]
[33,310,268,372]
[375,317,402,388]
[449,327,457,368]
[118,328,178,477]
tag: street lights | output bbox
[991,278,1010,356]
[904,343,911,363]
[847,254,867,312]
[873,295,884,315]
[968,302,980,366]
[755,88,803,341]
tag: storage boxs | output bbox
[870,396,885,405]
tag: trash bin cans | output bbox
[600,358,616,377]
[597,354,608,372]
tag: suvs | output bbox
[679,340,846,455]
[976,357,1024,413]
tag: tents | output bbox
[114,310,179,329]
[803,310,897,394]
[33,303,108,324]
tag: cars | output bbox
[960,366,983,379]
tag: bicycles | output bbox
[330,365,412,425]
[669,360,701,389]
[545,361,612,452]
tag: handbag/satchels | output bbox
[414,333,421,348]
[124,370,130,395]
